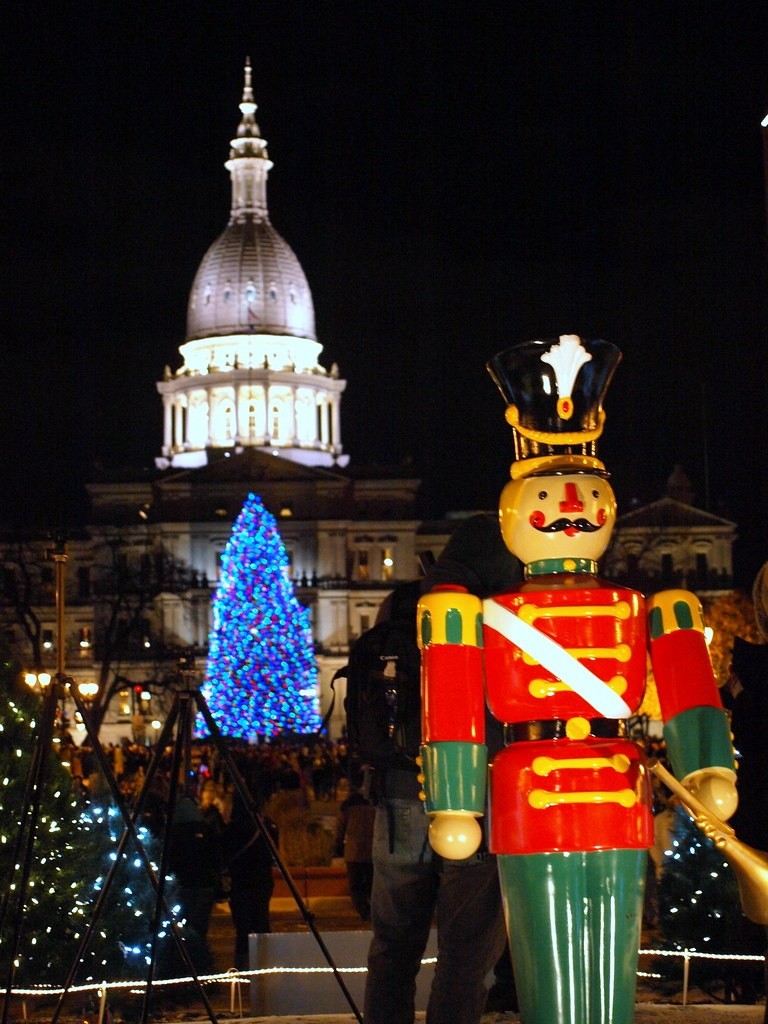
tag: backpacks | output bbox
[316,580,422,858]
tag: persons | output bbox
[55,737,333,803]
[416,334,738,1024]
[335,514,516,1024]
[135,776,276,977]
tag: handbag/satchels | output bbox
[214,867,233,902]
